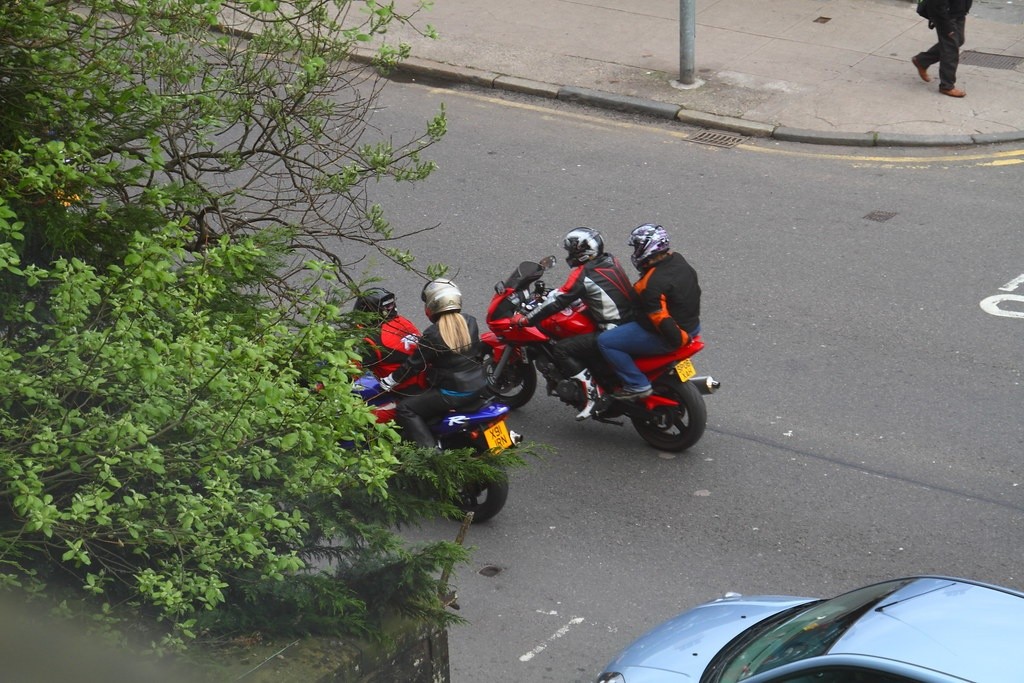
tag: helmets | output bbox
[563,227,603,268]
[628,224,670,270]
[354,287,396,325]
[421,278,462,322]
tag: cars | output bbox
[597,571,1024,683]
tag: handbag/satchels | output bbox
[917,0,936,29]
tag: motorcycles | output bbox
[479,258,721,452]
[321,380,524,525]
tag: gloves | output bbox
[510,314,524,328]
[379,373,400,392]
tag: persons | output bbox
[511,227,639,422]
[312,286,425,451]
[597,224,701,400]
[911,0,973,97]
[380,278,488,456]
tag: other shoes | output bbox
[912,57,931,83]
[611,385,653,398]
[939,87,966,97]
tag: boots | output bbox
[570,368,607,421]
